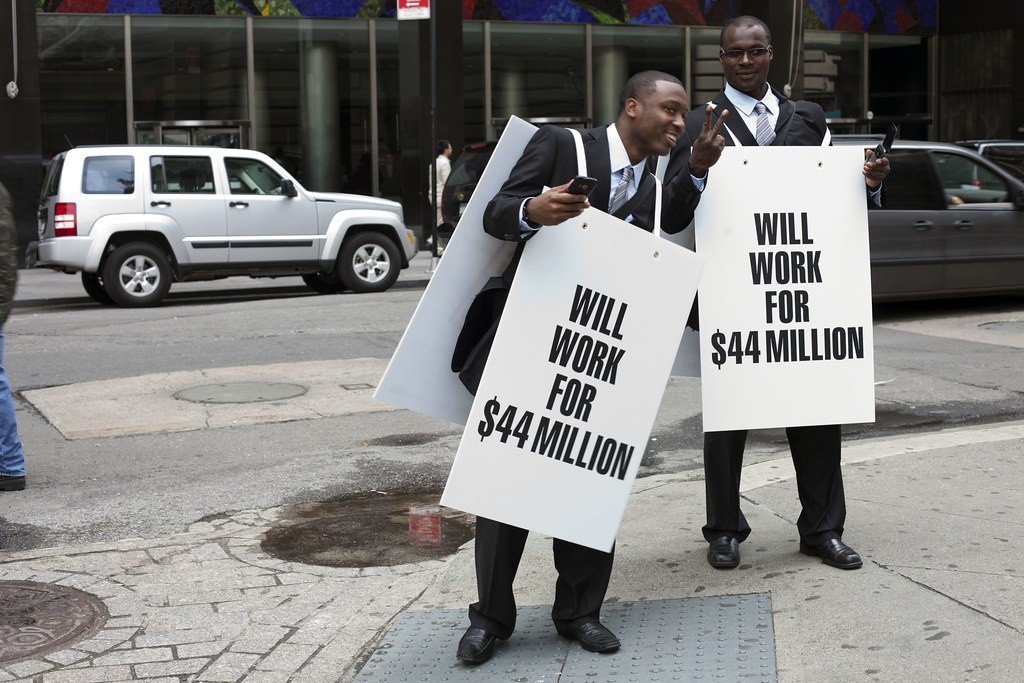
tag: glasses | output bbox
[720,44,771,58]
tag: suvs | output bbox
[36,144,419,309]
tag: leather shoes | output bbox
[707,536,741,570]
[457,624,497,667]
[800,534,863,571]
[555,617,622,654]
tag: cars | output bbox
[831,133,1024,304]
[927,140,1024,204]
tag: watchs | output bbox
[522,198,533,227]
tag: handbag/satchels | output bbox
[450,275,510,396]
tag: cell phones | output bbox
[566,176,598,197]
[867,122,897,163]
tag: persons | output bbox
[0,182,25,491]
[946,193,963,204]
[451,72,688,662]
[661,16,890,571]
[428,138,452,253]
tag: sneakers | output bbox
[0,468,27,492]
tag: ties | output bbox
[754,103,777,146]
[607,166,634,216]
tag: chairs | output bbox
[177,170,205,192]
